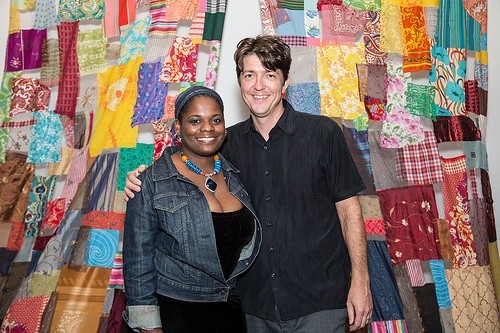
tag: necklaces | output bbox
[180,146,222,192]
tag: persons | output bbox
[123,86,261,333]
[123,33,373,333]
